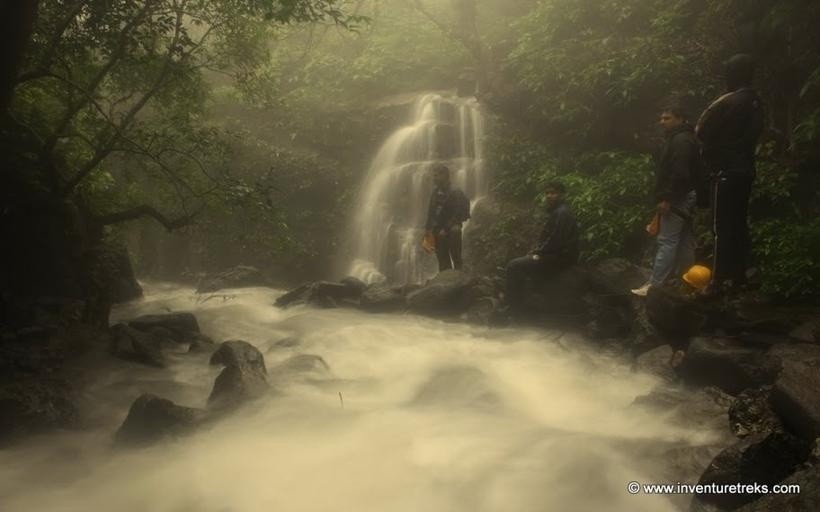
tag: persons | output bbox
[696,53,762,298]
[425,161,471,273]
[506,180,576,313]
[630,103,703,297]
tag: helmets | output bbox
[683,265,713,290]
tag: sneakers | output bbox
[630,283,652,298]
